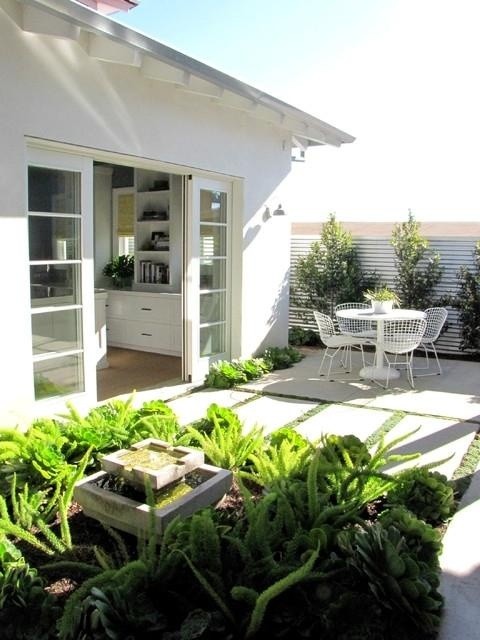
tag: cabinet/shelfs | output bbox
[30,165,221,356]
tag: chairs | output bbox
[313,301,448,391]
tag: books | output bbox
[150,231,169,251]
[139,260,169,284]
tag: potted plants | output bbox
[361,286,402,315]
